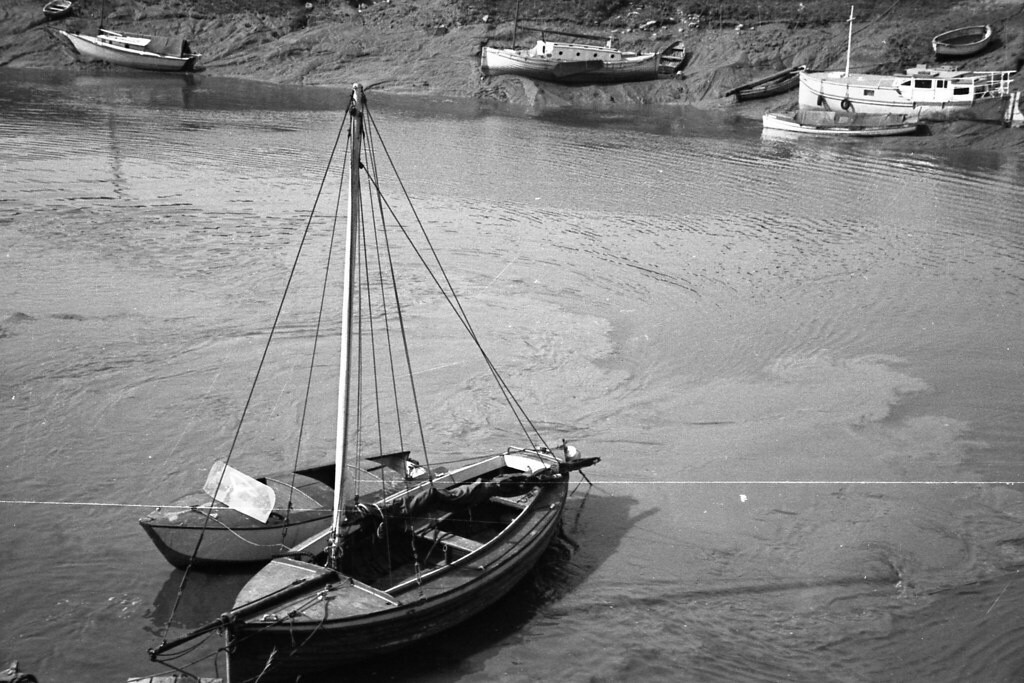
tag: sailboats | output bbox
[148,82,602,683]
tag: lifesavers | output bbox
[841,99,852,110]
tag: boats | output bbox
[481,0,661,81]
[44,0,202,72]
[791,5,1024,127]
[726,65,807,101]
[43,0,72,17]
[763,111,925,137]
[931,25,992,55]
[659,41,686,74]
[139,451,448,574]
